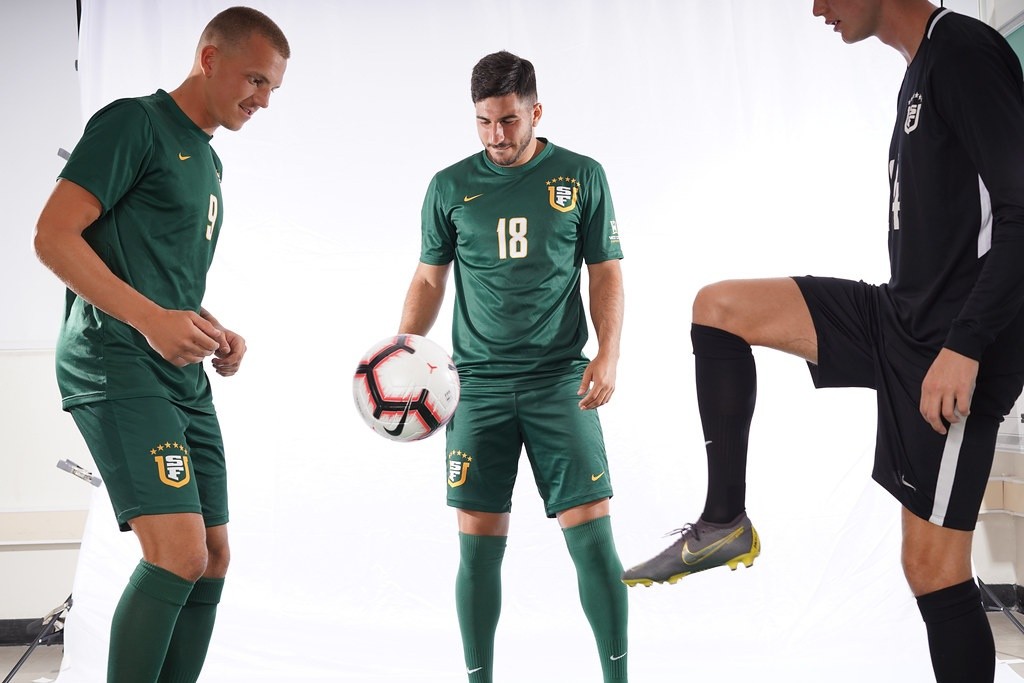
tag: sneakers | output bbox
[620,514,760,587]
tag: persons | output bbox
[34,6,291,683]
[400,50,628,683]
[622,0,1024,683]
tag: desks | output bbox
[0,508,92,683]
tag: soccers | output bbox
[351,332,462,444]
